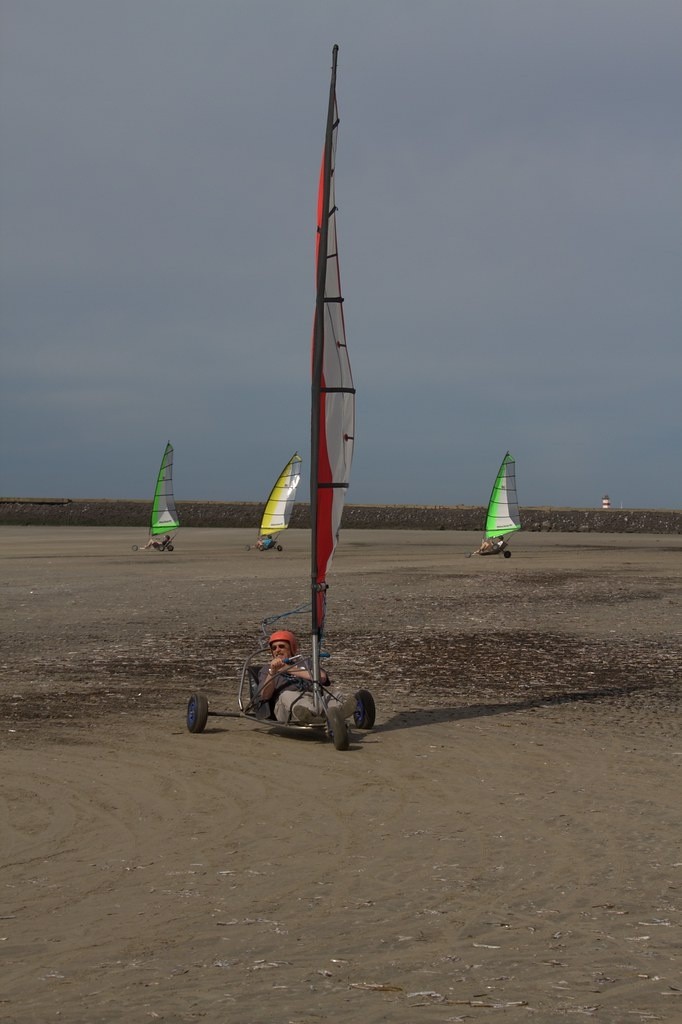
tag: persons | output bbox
[145,535,170,547]
[257,630,358,726]
[474,535,504,553]
[255,535,273,548]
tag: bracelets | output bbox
[268,668,276,676]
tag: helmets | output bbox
[268,631,297,656]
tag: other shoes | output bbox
[340,697,358,719]
[292,704,325,724]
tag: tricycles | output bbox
[465,450,522,559]
[132,439,181,552]
[245,450,304,552]
[186,44,378,750]
